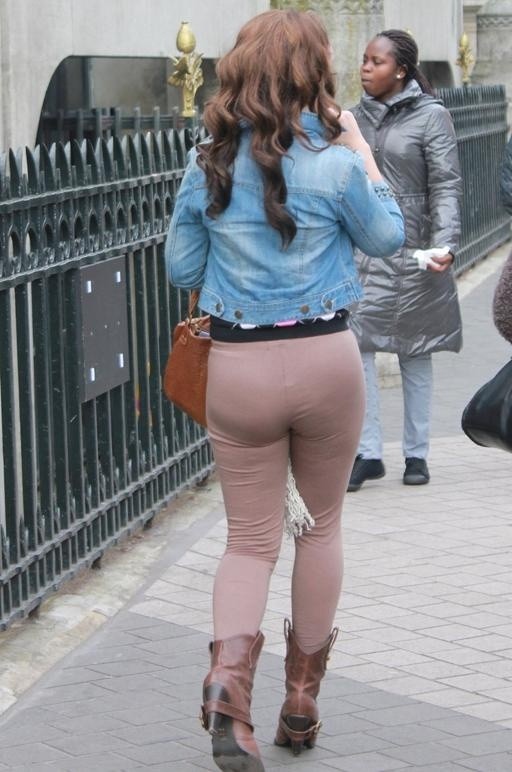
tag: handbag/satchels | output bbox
[163,310,212,428]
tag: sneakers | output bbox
[460,362,512,452]
[348,455,385,490]
[403,457,430,484]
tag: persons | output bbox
[161,6,405,772]
[342,28,464,492]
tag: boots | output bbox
[275,618,339,757]
[202,631,266,771]
